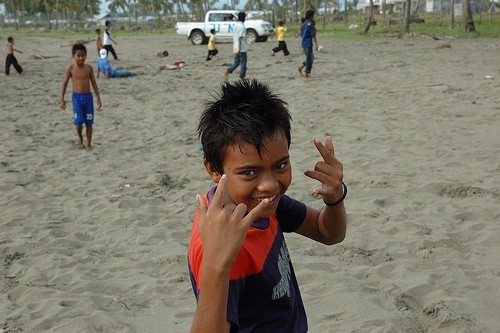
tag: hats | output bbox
[99,48,107,59]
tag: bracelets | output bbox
[323,181,347,206]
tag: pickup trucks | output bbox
[175,8,274,45]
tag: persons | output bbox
[187,79,347,333]
[96,48,137,80]
[94,29,103,57]
[103,20,118,60]
[223,12,247,81]
[298,10,318,80]
[4,37,23,75]
[271,21,290,56]
[59,44,102,150]
[206,29,219,61]
